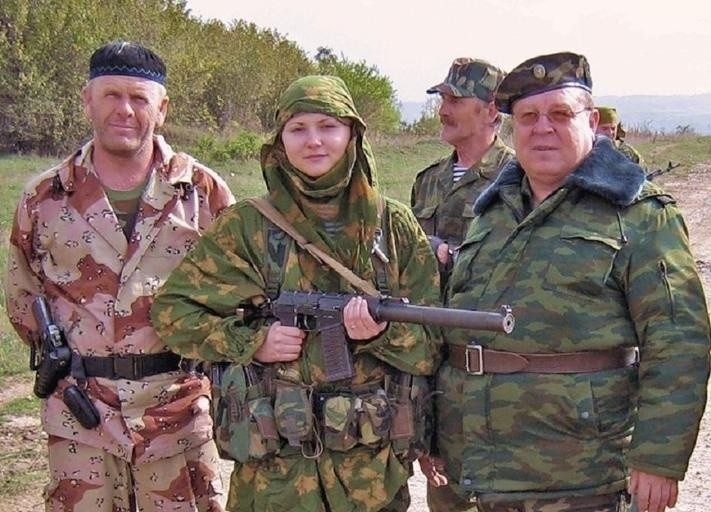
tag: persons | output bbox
[8,41,236,511]
[418,51,710,510]
[410,58,514,511]
[151,76,443,511]
[595,107,643,165]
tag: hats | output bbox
[89,43,167,89]
[427,57,508,101]
[595,105,617,125]
[495,52,593,115]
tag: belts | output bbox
[444,344,638,374]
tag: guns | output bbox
[30,297,64,398]
[236,289,516,385]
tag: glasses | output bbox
[508,103,592,125]
